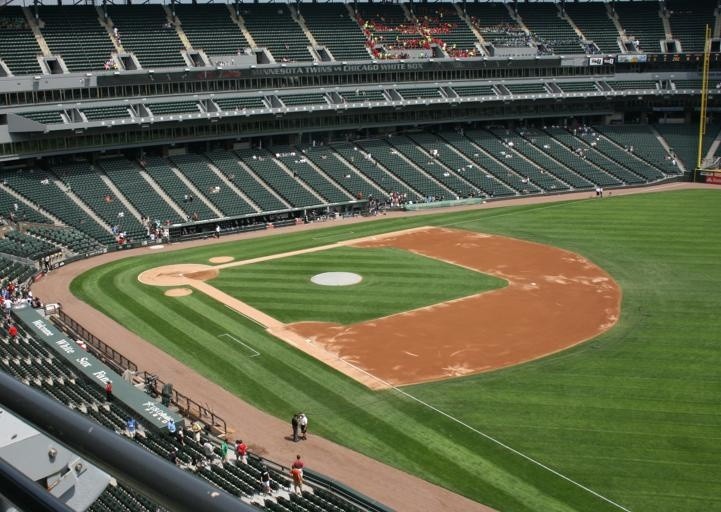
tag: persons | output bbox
[9,324,17,338]
[113,217,168,246]
[216,224,220,238]
[127,412,308,495]
[0,282,32,315]
[107,381,112,402]
